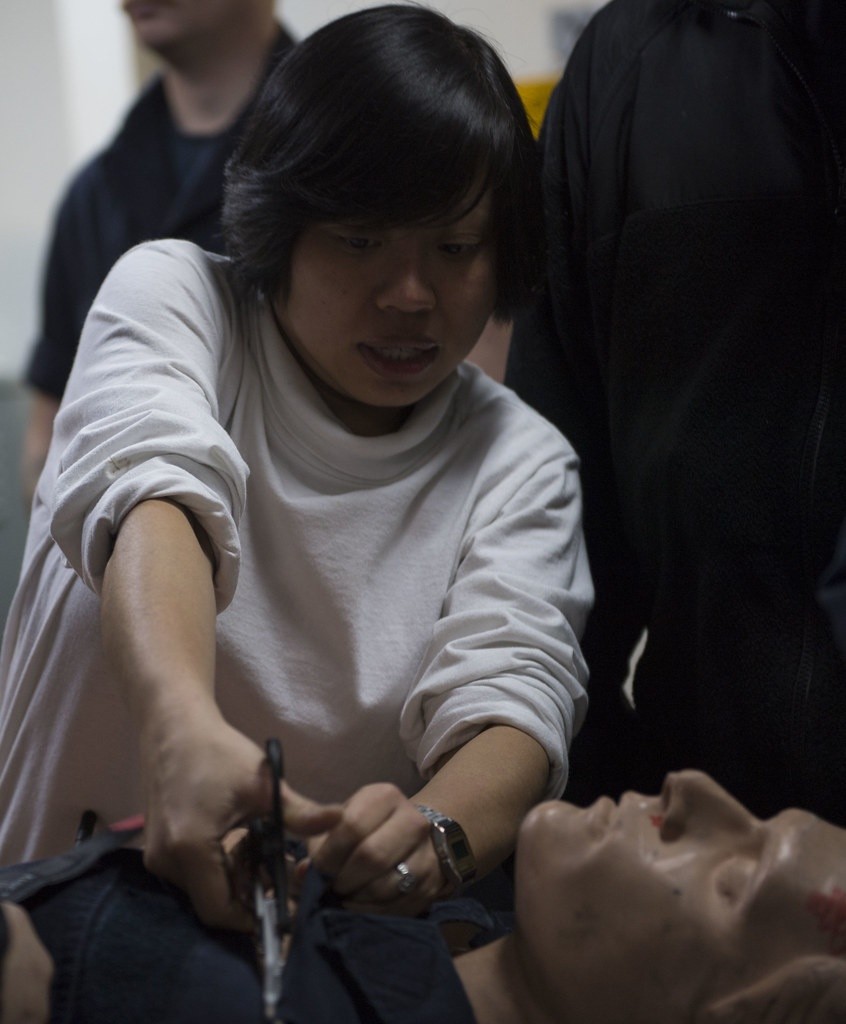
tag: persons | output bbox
[1,0,598,934]
[1,765,844,1024]
[13,0,305,517]
[504,1,845,828]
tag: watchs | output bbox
[411,803,483,893]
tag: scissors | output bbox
[244,734,292,1022]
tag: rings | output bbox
[397,861,411,895]
